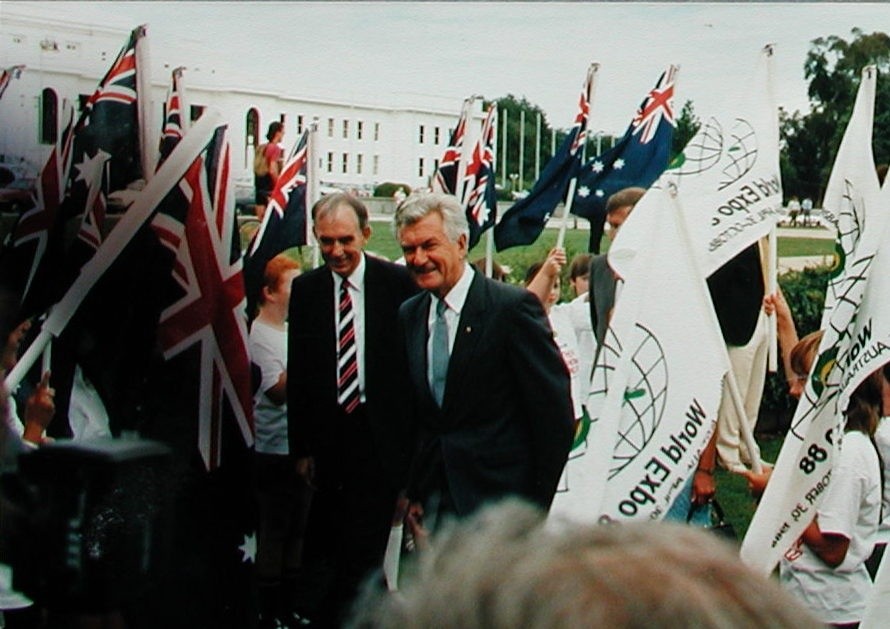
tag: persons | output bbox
[345,495,826,629]
[289,191,426,628]
[374,193,576,565]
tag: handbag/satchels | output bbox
[687,497,738,547]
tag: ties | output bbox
[431,299,452,409]
[337,278,360,413]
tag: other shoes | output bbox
[717,457,749,473]
[759,459,774,469]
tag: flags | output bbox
[417,44,890,629]
[0,26,322,507]
